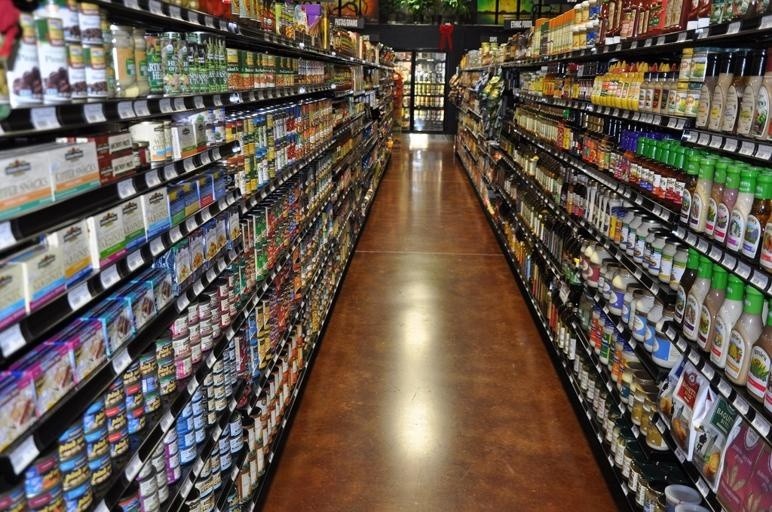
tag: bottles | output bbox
[494,0,771,512]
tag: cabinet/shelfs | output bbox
[413,49,447,133]
[394,50,413,132]
